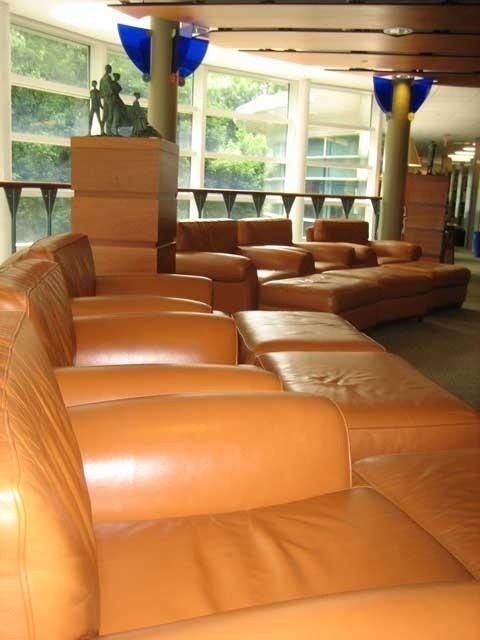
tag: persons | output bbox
[132,92,149,137]
[89,80,104,136]
[112,73,124,136]
[98,64,117,136]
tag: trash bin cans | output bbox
[453,227,466,247]
[472,232,480,258]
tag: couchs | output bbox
[0,220,480,639]
